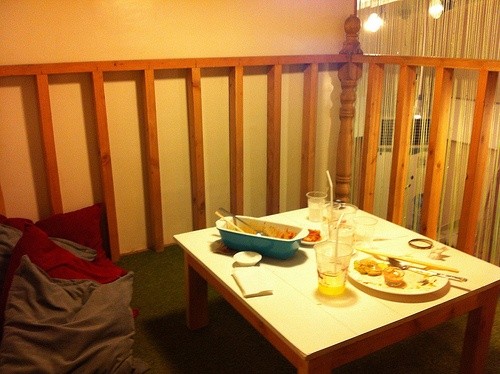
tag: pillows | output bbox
[0,226,97,273]
[0,255,151,374]
[0,226,140,330]
[0,203,137,374]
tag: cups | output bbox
[326,203,358,255]
[313,241,353,296]
[306,191,328,222]
[352,216,378,250]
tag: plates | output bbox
[347,249,450,296]
[300,227,329,245]
[232,251,262,266]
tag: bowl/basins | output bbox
[215,216,309,261]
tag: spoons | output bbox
[219,207,268,236]
[388,257,468,282]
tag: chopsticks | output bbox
[355,247,461,273]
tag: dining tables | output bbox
[171,202,499,374]
